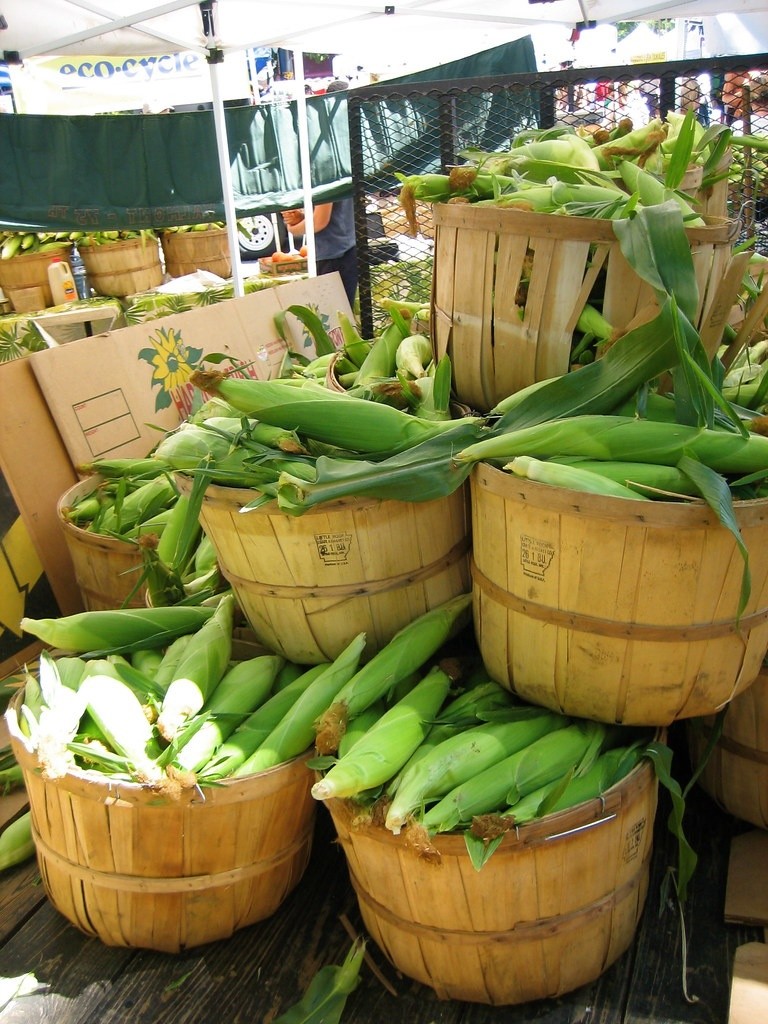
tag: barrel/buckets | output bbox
[0,201,768,1005]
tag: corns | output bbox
[0,109,768,873]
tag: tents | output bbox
[0,1,768,299]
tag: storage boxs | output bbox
[258,253,308,274]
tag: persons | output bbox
[281,197,358,309]
[558,69,768,143]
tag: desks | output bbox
[0,270,308,362]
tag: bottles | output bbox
[71,247,89,299]
[48,257,78,306]
